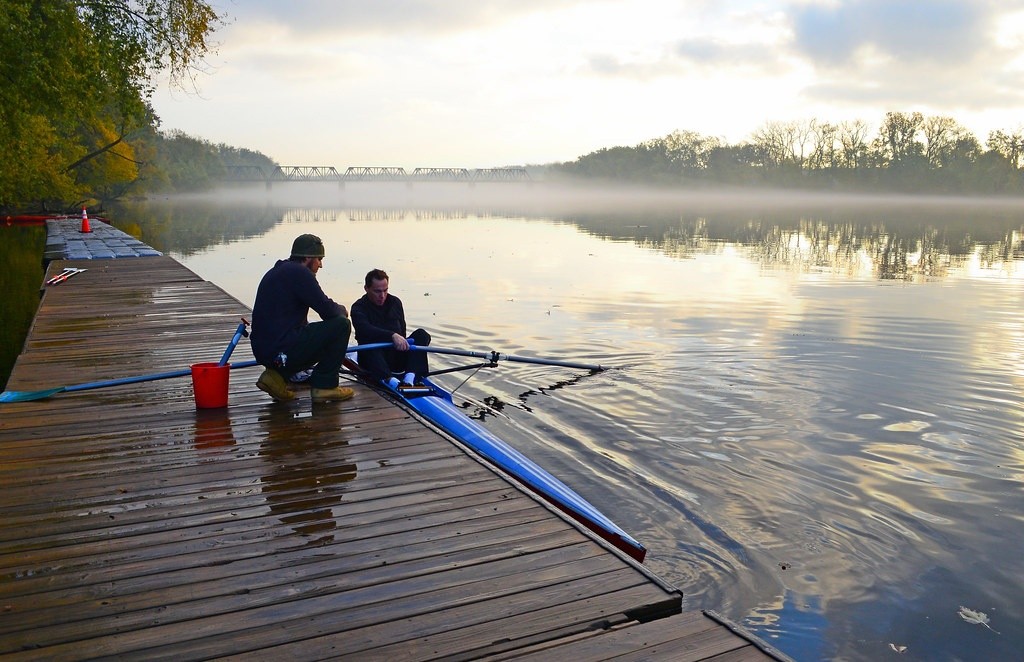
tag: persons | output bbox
[349,268,432,396]
[248,233,355,405]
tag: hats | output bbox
[291,234,325,257]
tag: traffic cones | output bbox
[78,206,93,234]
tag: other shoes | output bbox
[388,377,400,389]
[402,373,415,386]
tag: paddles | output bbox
[408,343,610,370]
[0,338,415,402]
[46,267,88,285]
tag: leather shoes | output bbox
[256,368,295,401]
[311,387,354,401]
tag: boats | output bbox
[349,342,645,566]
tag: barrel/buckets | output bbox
[190,361,232,408]
